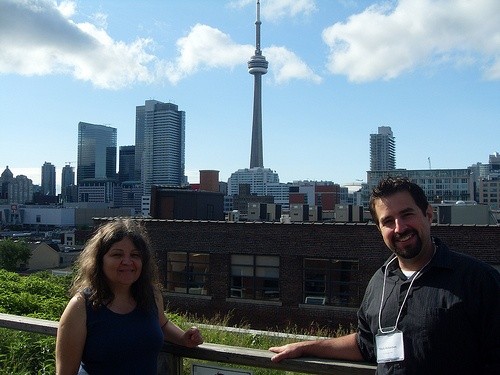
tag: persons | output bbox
[269,175,500,375]
[54,218,204,375]
